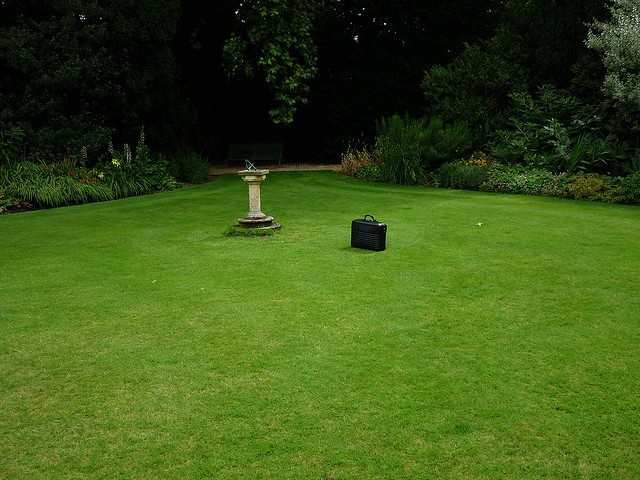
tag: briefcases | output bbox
[351,214,387,251]
